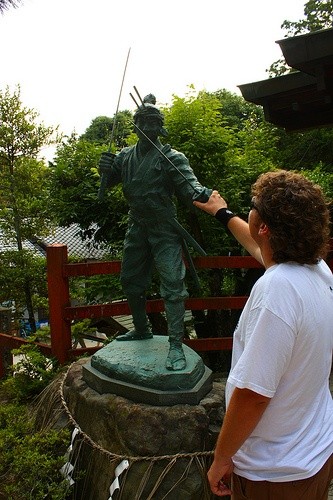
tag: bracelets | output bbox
[215,207,235,227]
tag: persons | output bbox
[192,170,333,500]
[99,93,215,371]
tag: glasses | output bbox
[248,201,261,218]
